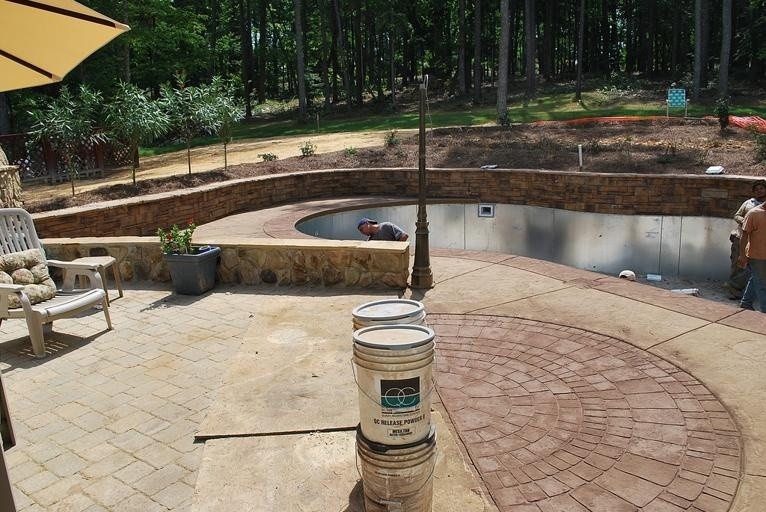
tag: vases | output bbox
[162,246,221,295]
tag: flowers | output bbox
[154,218,196,257]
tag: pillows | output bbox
[0,248,56,309]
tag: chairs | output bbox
[0,208,113,358]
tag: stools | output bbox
[62,256,123,307]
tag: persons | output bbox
[357,217,410,241]
[738,201,766,313]
[722,179,766,300]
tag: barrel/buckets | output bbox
[355,421,438,512]
[350,299,427,333]
[352,324,434,445]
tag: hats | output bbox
[357,218,377,229]
[619,270,636,281]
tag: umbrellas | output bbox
[0,0,131,98]
